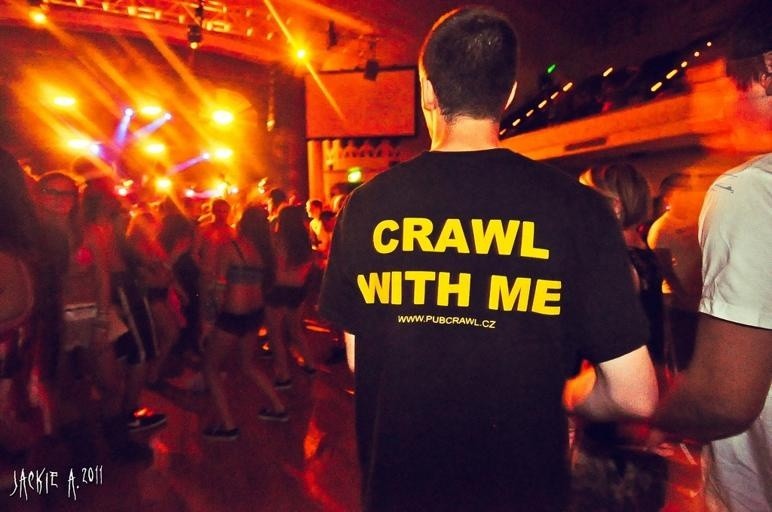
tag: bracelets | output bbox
[98,303,109,315]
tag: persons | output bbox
[201,208,292,441]
[317,211,336,269]
[306,198,329,251]
[155,194,200,323]
[80,176,170,433]
[331,194,345,213]
[191,201,237,336]
[39,172,154,487]
[126,174,304,217]
[315,8,660,512]
[647,174,698,388]
[266,206,318,389]
[649,30,771,512]
[129,212,188,352]
[573,162,667,512]
[0,146,68,512]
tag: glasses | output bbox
[41,187,73,197]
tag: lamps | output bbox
[187,0,204,50]
[361,38,386,82]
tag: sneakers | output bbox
[300,364,319,378]
[127,411,168,433]
[258,406,290,422]
[272,375,293,390]
[202,423,241,441]
